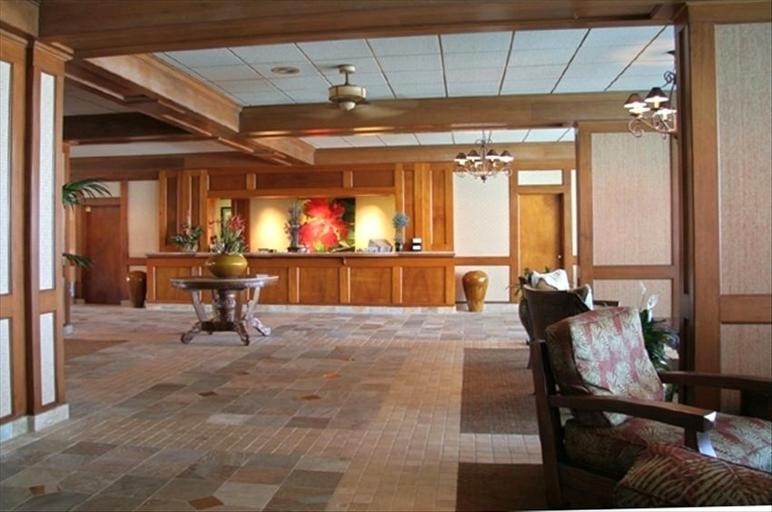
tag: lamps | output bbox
[622,61,679,140]
[452,127,515,184]
[328,64,367,110]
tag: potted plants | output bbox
[506,265,553,344]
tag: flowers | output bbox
[206,215,250,256]
[167,221,204,249]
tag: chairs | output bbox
[519,269,771,505]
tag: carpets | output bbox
[455,347,552,511]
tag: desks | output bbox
[169,272,279,347]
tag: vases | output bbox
[179,242,200,252]
[205,251,249,278]
[124,271,146,310]
[462,270,488,313]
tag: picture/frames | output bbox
[220,205,232,238]
